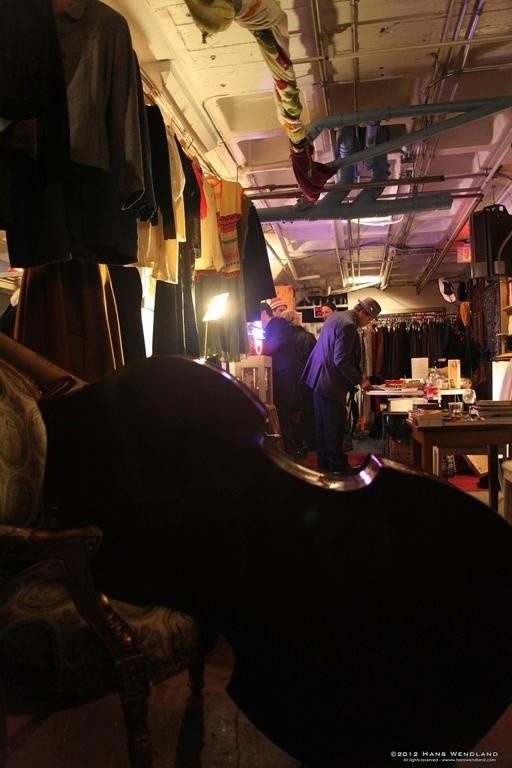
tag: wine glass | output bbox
[462,390,478,423]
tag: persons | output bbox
[253,302,298,454]
[281,309,319,449]
[267,296,288,319]
[303,297,382,477]
[320,302,338,320]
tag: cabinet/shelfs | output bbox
[495,275,512,360]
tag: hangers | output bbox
[356,310,474,334]
[164,117,242,186]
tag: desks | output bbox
[356,378,512,527]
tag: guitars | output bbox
[36,354,511,766]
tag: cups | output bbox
[448,401,464,422]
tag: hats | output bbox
[358,296,381,322]
[270,297,288,310]
